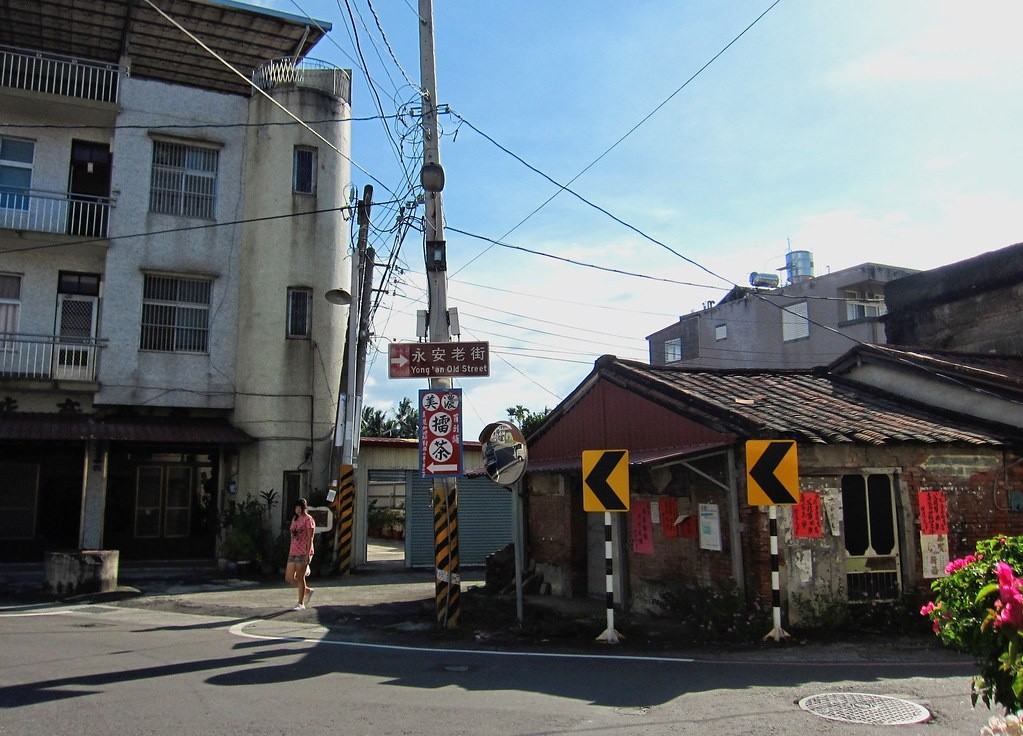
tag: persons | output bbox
[285,498,316,611]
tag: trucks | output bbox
[512,441,525,460]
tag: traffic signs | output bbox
[387,342,491,378]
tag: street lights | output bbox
[419,164,460,632]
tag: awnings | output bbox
[0,411,238,443]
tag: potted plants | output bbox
[214,490,281,576]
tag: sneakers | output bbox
[290,603,306,611]
[304,589,313,604]
